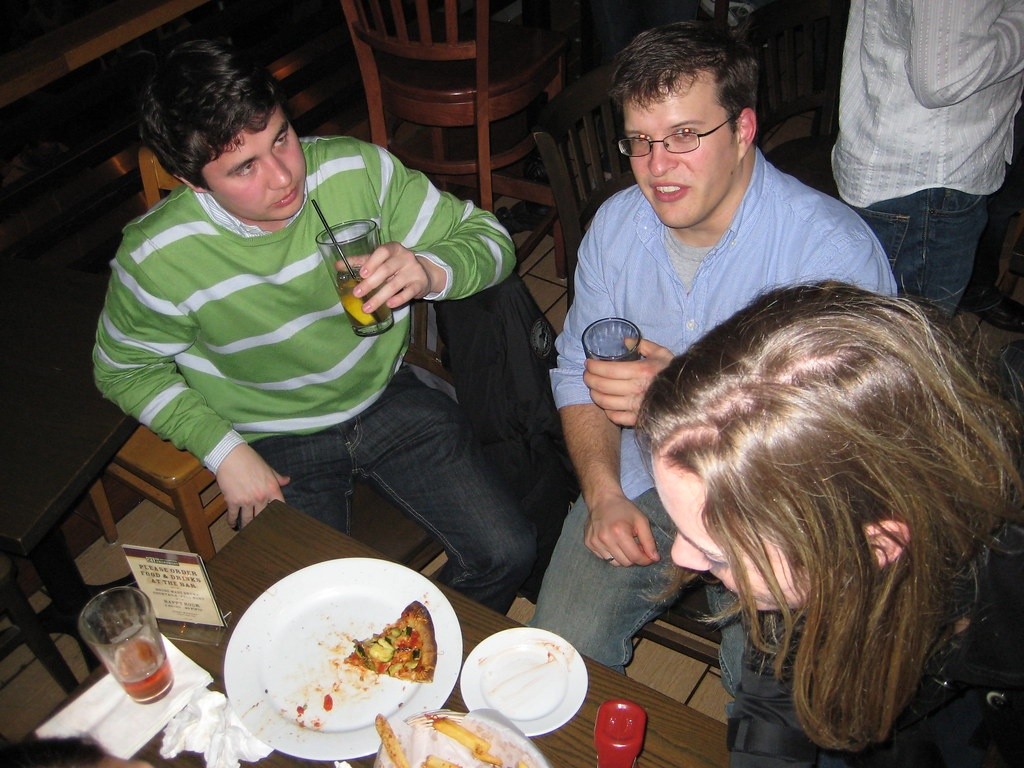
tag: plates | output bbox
[223,557,464,761]
[459,627,589,737]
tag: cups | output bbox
[582,317,641,362]
[314,218,394,337]
[78,585,175,705]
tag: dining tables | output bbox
[28,501,730,768]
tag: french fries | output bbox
[375,713,535,768]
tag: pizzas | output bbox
[344,600,438,684]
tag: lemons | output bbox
[338,273,391,325]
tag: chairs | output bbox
[0,1,842,562]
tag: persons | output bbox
[0,737,154,768]
[635,280,1024,768]
[93,31,541,611]
[828,0,1024,314]
[527,20,897,714]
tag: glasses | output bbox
[611,103,747,157]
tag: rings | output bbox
[606,557,614,562]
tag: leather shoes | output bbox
[971,294,1024,333]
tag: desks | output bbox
[0,268,140,696]
